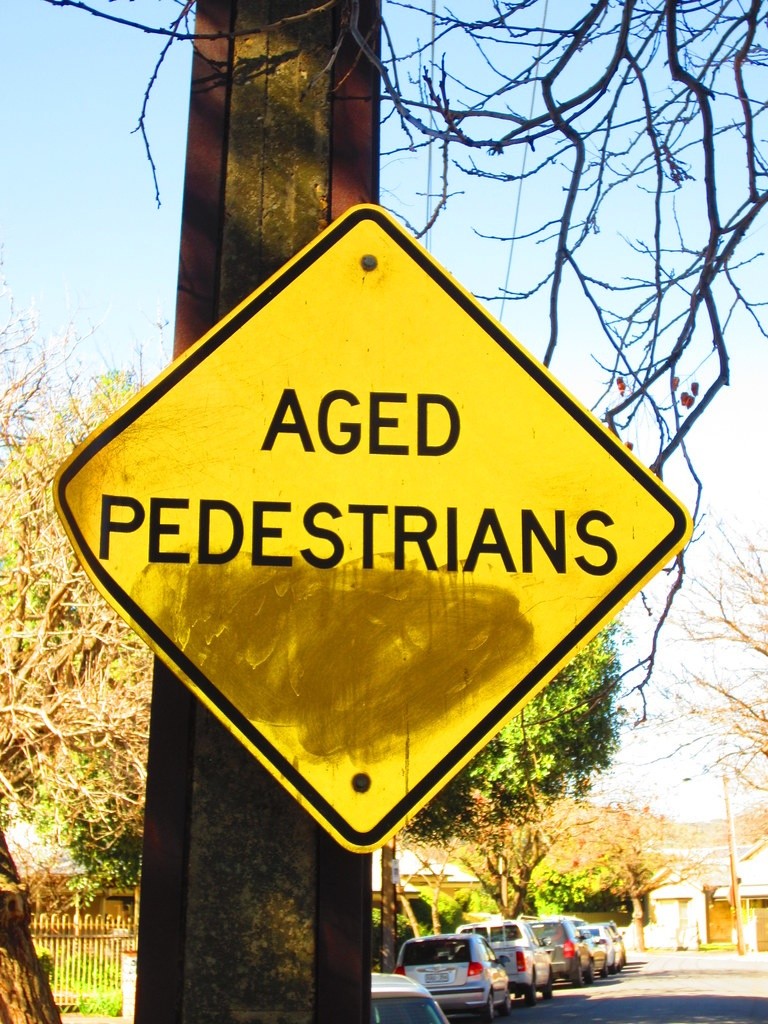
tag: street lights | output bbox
[682,769,745,957]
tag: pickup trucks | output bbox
[455,918,555,1007]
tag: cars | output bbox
[364,972,451,1024]
[392,933,512,1024]
[516,914,627,988]
